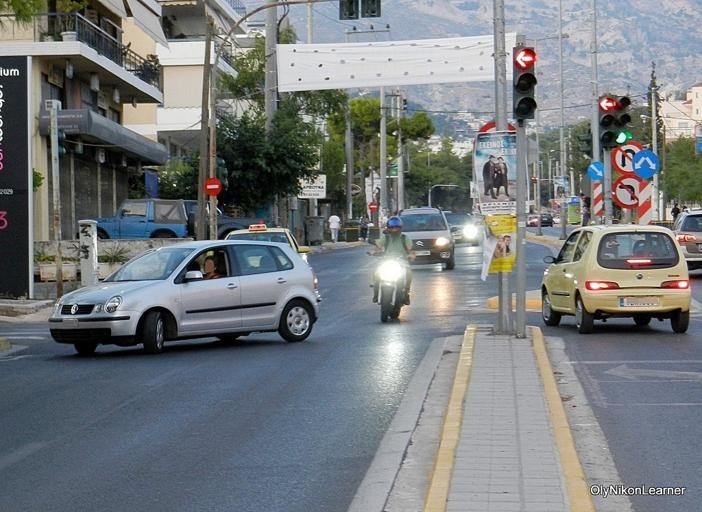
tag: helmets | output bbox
[386,216,402,228]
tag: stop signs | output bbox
[203,177,222,196]
[368,201,378,212]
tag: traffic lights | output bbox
[512,45,537,121]
[598,94,633,148]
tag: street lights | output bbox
[428,184,459,207]
[416,149,440,206]
[640,114,660,223]
[539,148,561,213]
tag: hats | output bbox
[489,155,504,161]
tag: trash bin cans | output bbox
[305,215,325,246]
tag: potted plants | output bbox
[56,0,89,42]
[38,254,80,282]
[98,244,132,280]
[38,23,55,42]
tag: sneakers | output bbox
[485,193,509,199]
[373,295,378,302]
[404,294,410,305]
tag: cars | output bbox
[47,223,323,355]
[539,223,691,335]
[527,212,553,228]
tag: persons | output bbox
[328,212,342,243]
[482,155,497,199]
[498,235,511,257]
[671,204,680,222]
[361,213,369,240]
[373,217,416,305]
[494,157,509,196]
[580,193,590,207]
[201,256,222,279]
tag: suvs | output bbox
[382,205,455,270]
[443,206,484,246]
[671,205,702,271]
[76,196,278,240]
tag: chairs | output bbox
[187,256,276,280]
[637,237,664,256]
[632,239,645,255]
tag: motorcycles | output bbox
[366,237,424,323]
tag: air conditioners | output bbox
[44,99,61,111]
